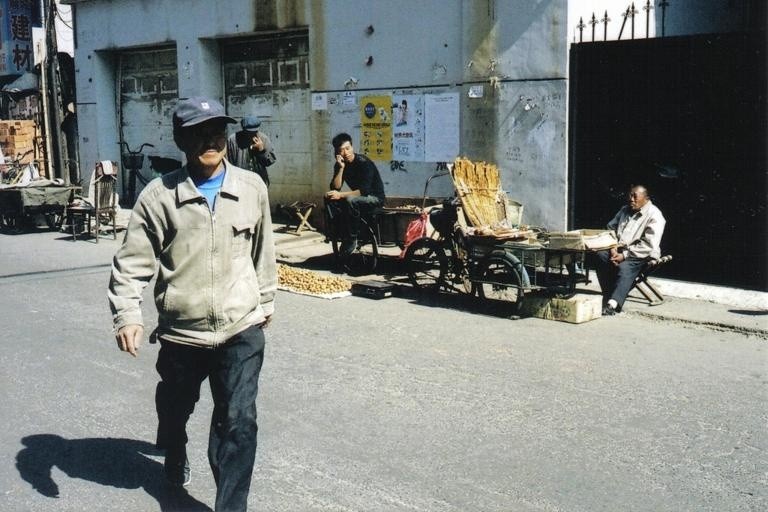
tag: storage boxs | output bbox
[518,228,617,324]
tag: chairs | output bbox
[66,159,119,245]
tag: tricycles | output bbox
[322,193,454,276]
[403,195,596,318]
[0,149,77,235]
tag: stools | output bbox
[627,254,673,307]
[276,199,316,236]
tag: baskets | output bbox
[123,153,144,169]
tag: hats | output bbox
[241,118,261,132]
[173,96,238,129]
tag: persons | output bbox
[224,113,276,187]
[323,132,387,275]
[595,185,667,313]
[108,97,278,511]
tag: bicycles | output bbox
[114,139,150,209]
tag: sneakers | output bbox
[602,304,615,315]
[165,454,192,486]
